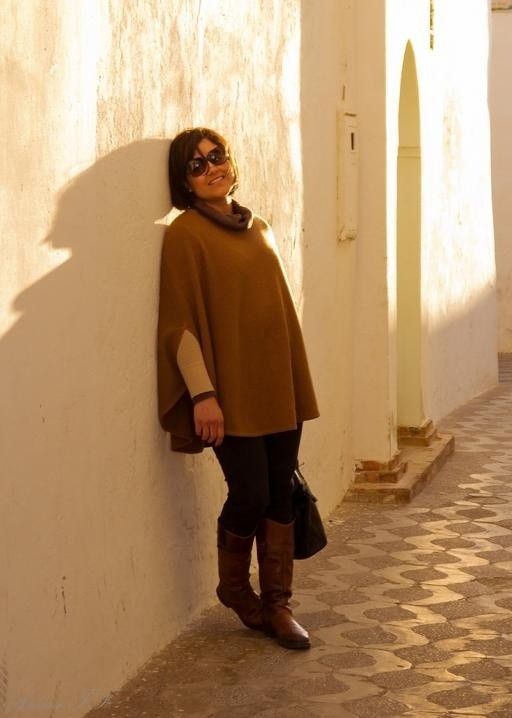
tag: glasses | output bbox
[186,146,228,177]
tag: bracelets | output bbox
[192,390,218,406]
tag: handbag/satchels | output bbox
[293,469,327,559]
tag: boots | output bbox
[216,516,262,630]
[256,517,311,650]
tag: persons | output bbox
[164,125,314,649]
